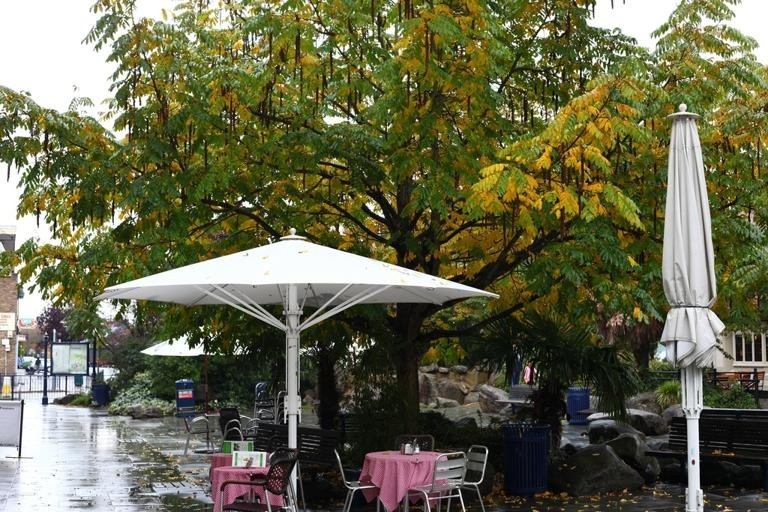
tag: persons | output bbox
[34,357,41,373]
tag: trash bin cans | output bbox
[566,387,590,425]
[501,424,551,495]
[92,384,105,407]
[175,379,195,417]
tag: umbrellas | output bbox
[97,226,501,510]
[658,101,726,512]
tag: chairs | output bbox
[178,381,302,512]
[332,434,489,512]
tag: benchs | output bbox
[251,414,401,479]
[645,407,768,492]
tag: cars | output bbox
[17,356,50,373]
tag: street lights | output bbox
[41,332,50,404]
